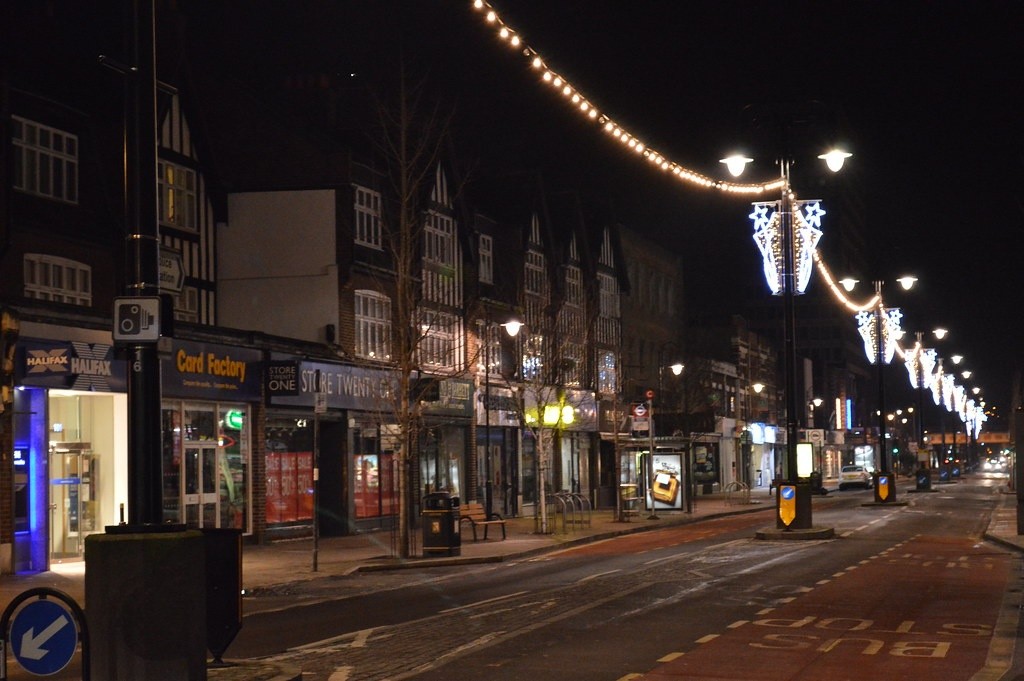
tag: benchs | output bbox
[460,504,507,542]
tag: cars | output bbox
[837,465,874,491]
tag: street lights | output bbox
[658,341,687,438]
[898,326,987,495]
[744,381,765,487]
[486,304,527,521]
[714,98,855,542]
[838,251,920,507]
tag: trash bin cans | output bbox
[810,471,820,495]
[422,491,461,558]
